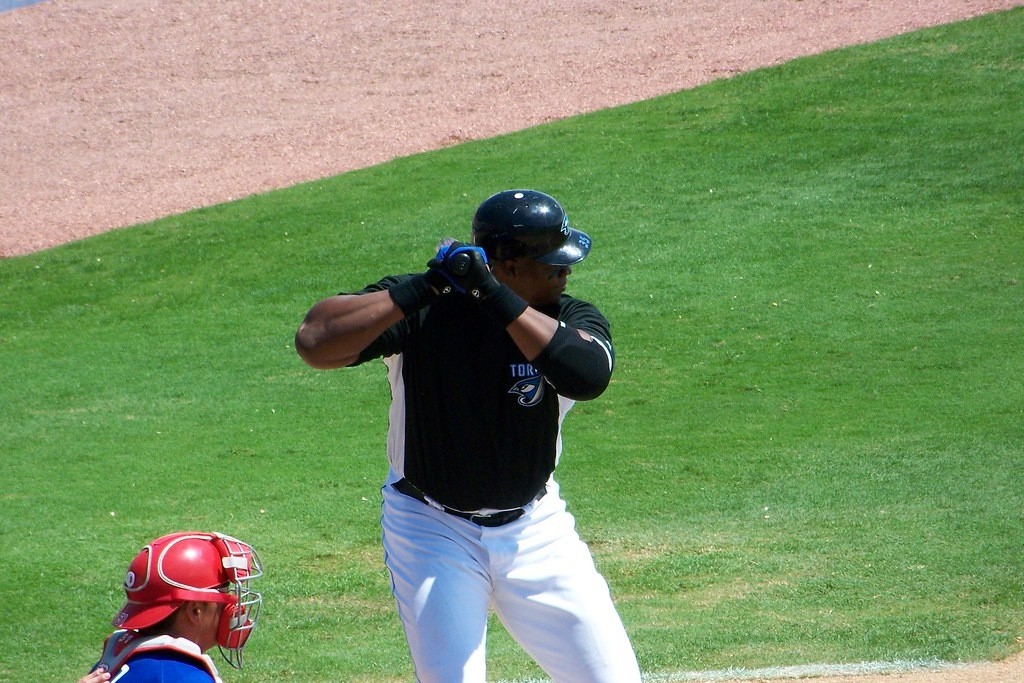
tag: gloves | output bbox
[389,241,529,329]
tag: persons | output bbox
[87,532,263,683]
[295,189,642,683]
[77,666,112,683]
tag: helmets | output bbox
[112,532,227,629]
[472,189,591,266]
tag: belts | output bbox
[392,478,547,527]
